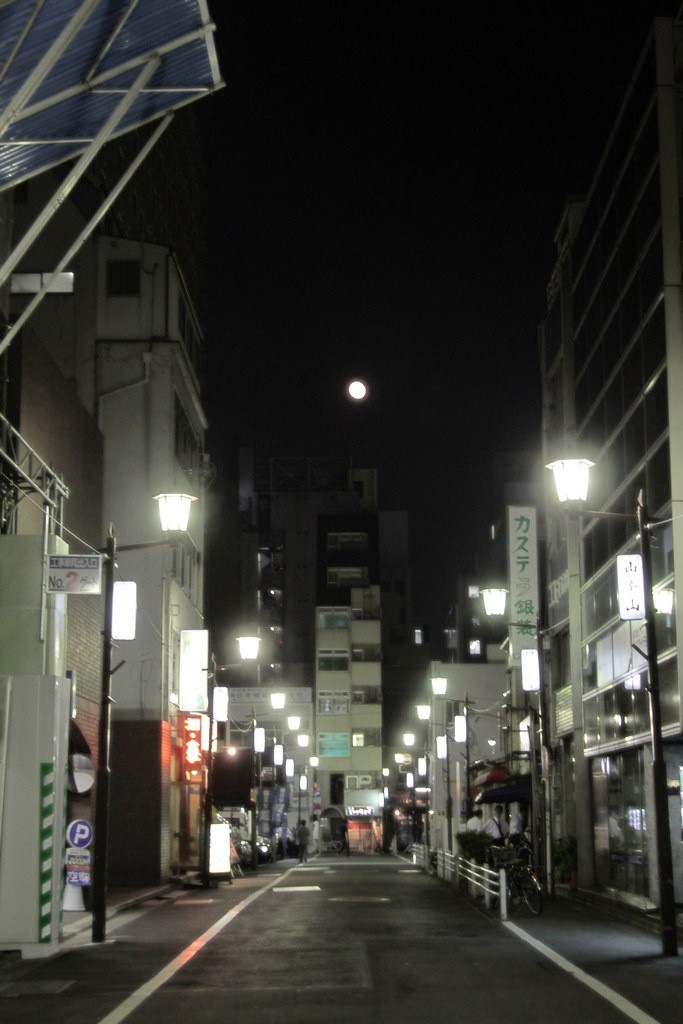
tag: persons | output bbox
[296,819,310,864]
[312,814,319,854]
[467,808,483,834]
[509,802,524,844]
[336,819,351,858]
[608,807,625,854]
[484,804,510,871]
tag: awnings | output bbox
[474,781,533,804]
[473,767,507,784]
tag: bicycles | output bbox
[490,844,545,917]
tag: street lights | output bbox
[270,713,302,865]
[541,442,679,958]
[244,692,286,875]
[413,702,454,855]
[199,631,262,889]
[280,734,308,862]
[429,672,476,819]
[478,586,546,847]
[295,755,320,858]
[86,487,200,945]
